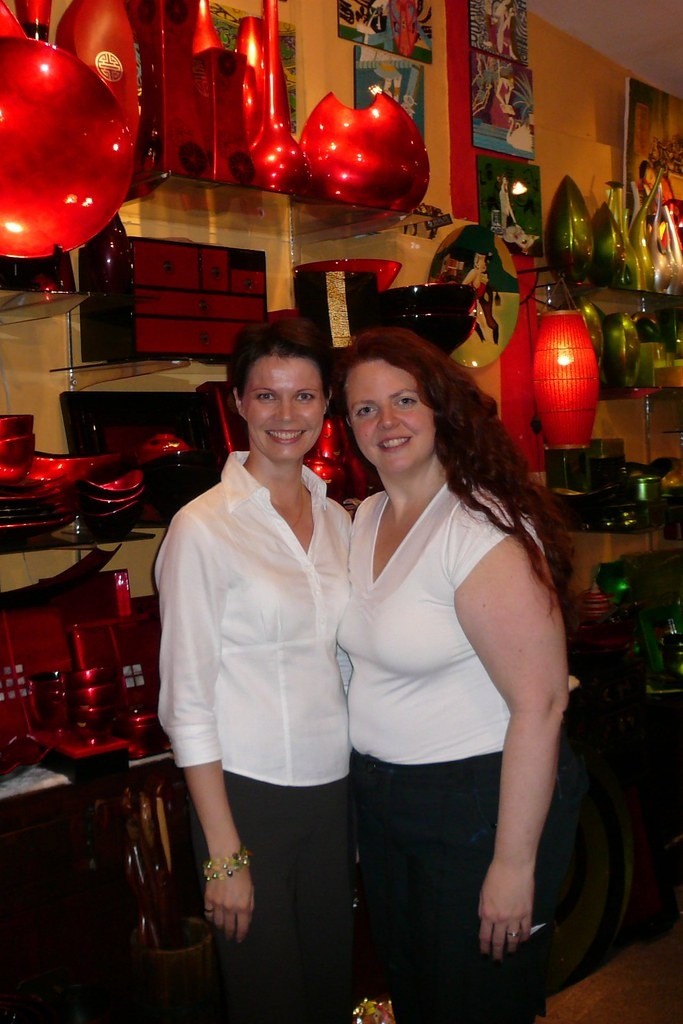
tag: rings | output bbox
[508,933,520,936]
[205,909,213,912]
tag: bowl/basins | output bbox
[74,470,145,544]
[0,413,35,483]
[66,667,121,737]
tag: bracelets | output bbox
[202,846,251,880]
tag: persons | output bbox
[154,320,351,1024]
[640,160,683,249]
[338,327,570,1023]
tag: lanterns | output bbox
[533,311,599,449]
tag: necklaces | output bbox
[291,487,304,528]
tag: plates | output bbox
[0,454,122,540]
